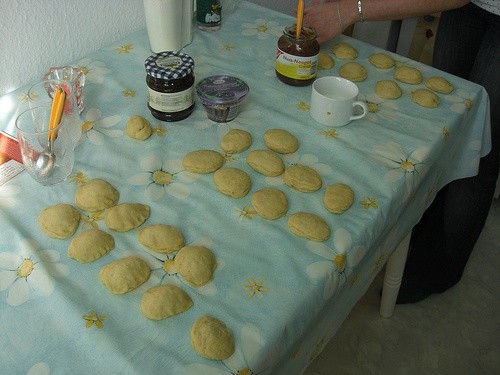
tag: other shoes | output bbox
[378,269,434,304]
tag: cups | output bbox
[310,76,369,127]
[27,79,82,150]
[15,106,75,185]
[42,66,86,116]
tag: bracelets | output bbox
[357,0,364,23]
[337,0,344,32]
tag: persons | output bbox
[293,0,500,305]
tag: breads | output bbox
[126,115,152,140]
[38,179,235,362]
[317,42,453,108]
[182,129,352,242]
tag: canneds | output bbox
[275,23,321,87]
[145,50,195,122]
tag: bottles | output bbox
[195,0,221,32]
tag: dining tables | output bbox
[0,0,493,375]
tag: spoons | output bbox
[36,88,67,180]
